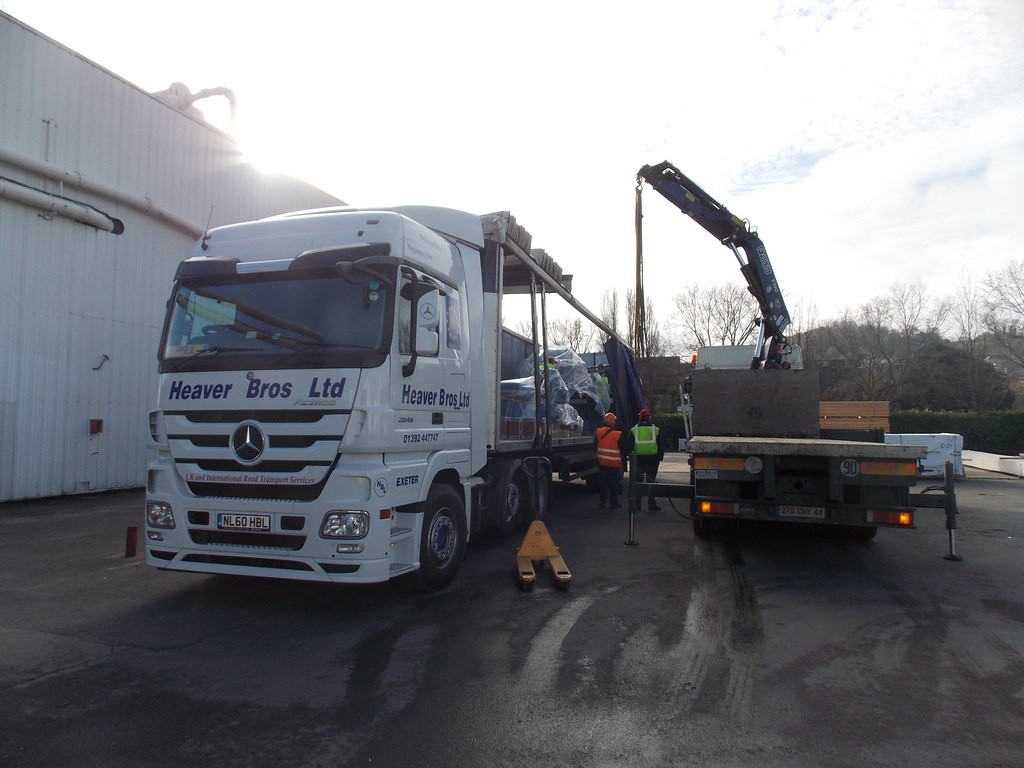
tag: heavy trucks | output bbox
[145,205,635,596]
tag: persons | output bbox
[593,408,665,512]
[530,358,555,376]
[596,363,608,398]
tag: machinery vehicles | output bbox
[633,159,929,541]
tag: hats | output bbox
[603,413,616,426]
[598,364,604,368]
[639,409,651,416]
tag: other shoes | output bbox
[600,502,607,507]
[648,505,661,511]
[635,503,641,510]
[611,503,623,509]
